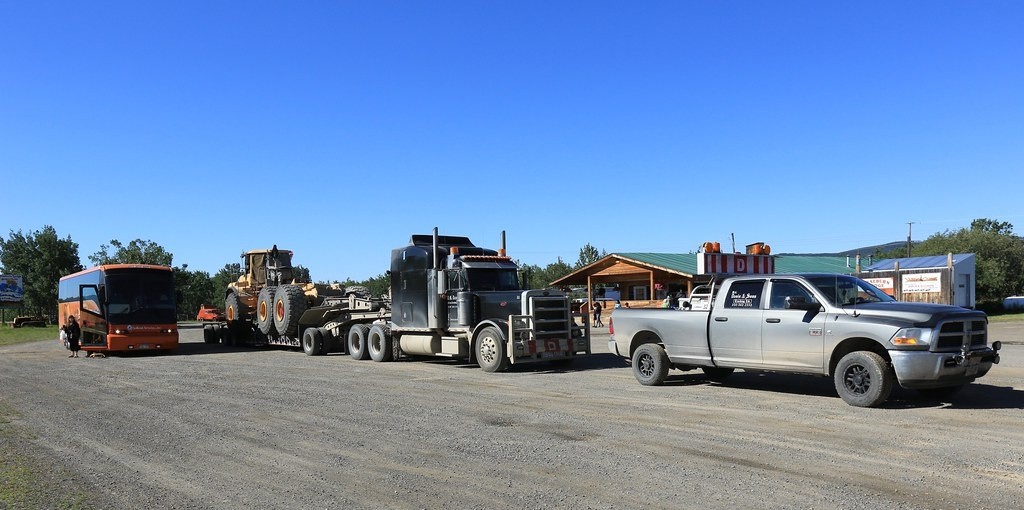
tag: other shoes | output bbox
[74,356,77,358]
[595,326,597,327]
[592,326,595,327]
[69,355,73,357]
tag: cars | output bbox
[197,308,226,322]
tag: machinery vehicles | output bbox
[225,244,371,336]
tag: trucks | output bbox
[678,283,818,311]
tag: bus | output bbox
[58,265,183,356]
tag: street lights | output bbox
[904,221,915,240]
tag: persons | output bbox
[666,293,673,308]
[625,302,630,309]
[62,315,80,358]
[595,302,604,328]
[592,303,600,327]
[0,278,22,295]
[676,289,686,298]
[84,332,103,358]
[479,271,497,290]
[615,300,621,309]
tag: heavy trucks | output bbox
[204,227,591,373]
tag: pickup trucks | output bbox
[609,273,1001,408]
